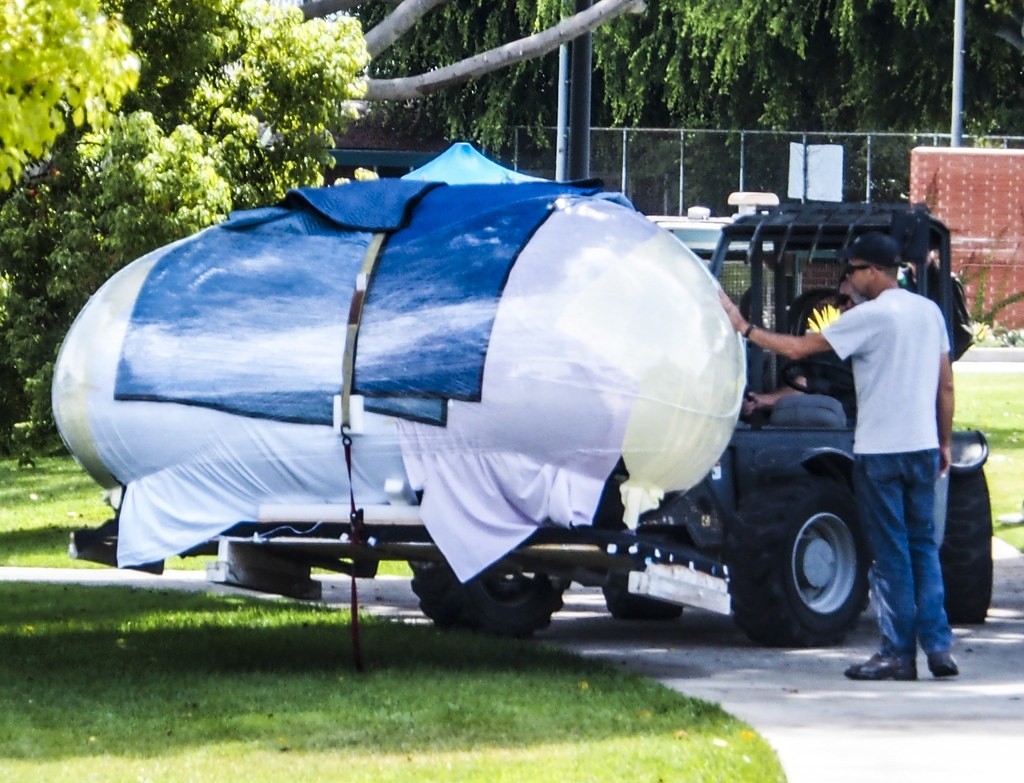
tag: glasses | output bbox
[844,264,882,273]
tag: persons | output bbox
[717,236,961,681]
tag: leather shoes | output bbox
[845,652,917,681]
[928,651,959,676]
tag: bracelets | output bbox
[743,322,757,337]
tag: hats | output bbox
[832,231,907,267]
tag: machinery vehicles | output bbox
[68,192,996,649]
